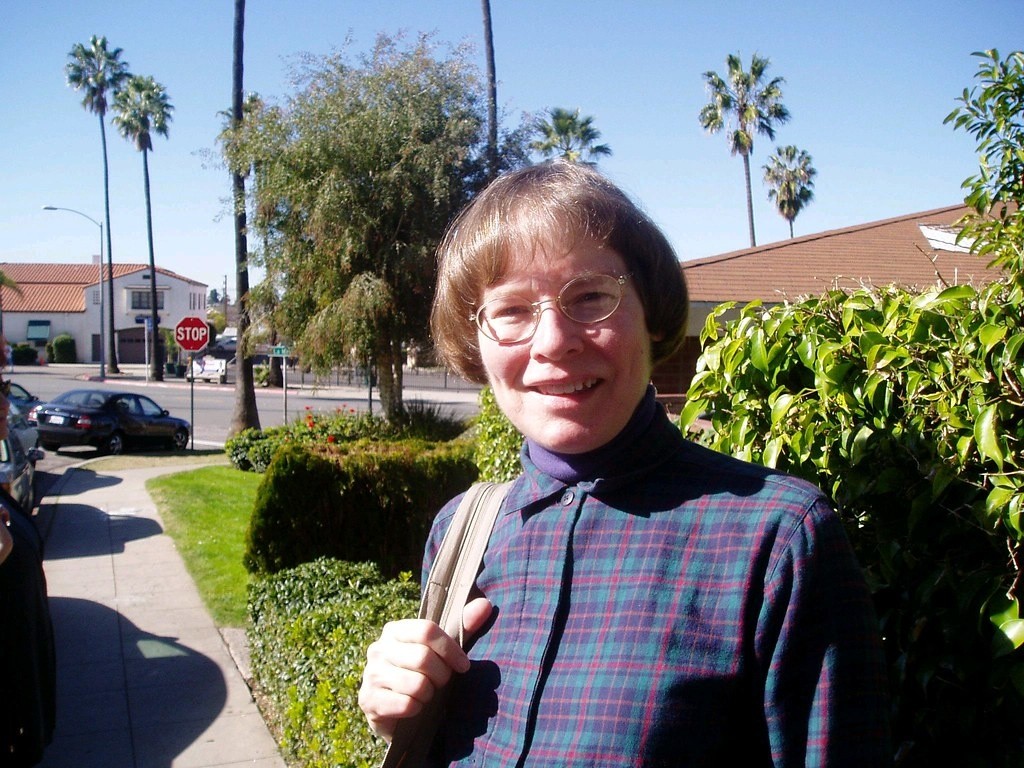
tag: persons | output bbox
[0,340,59,768]
[356,165,898,768]
[406,339,420,376]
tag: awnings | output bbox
[26,325,49,339]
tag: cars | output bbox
[0,380,61,765]
[34,389,191,455]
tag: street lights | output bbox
[42,205,107,383]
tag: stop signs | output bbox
[173,317,210,353]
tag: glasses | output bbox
[469,270,636,344]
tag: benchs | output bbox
[184,359,227,384]
